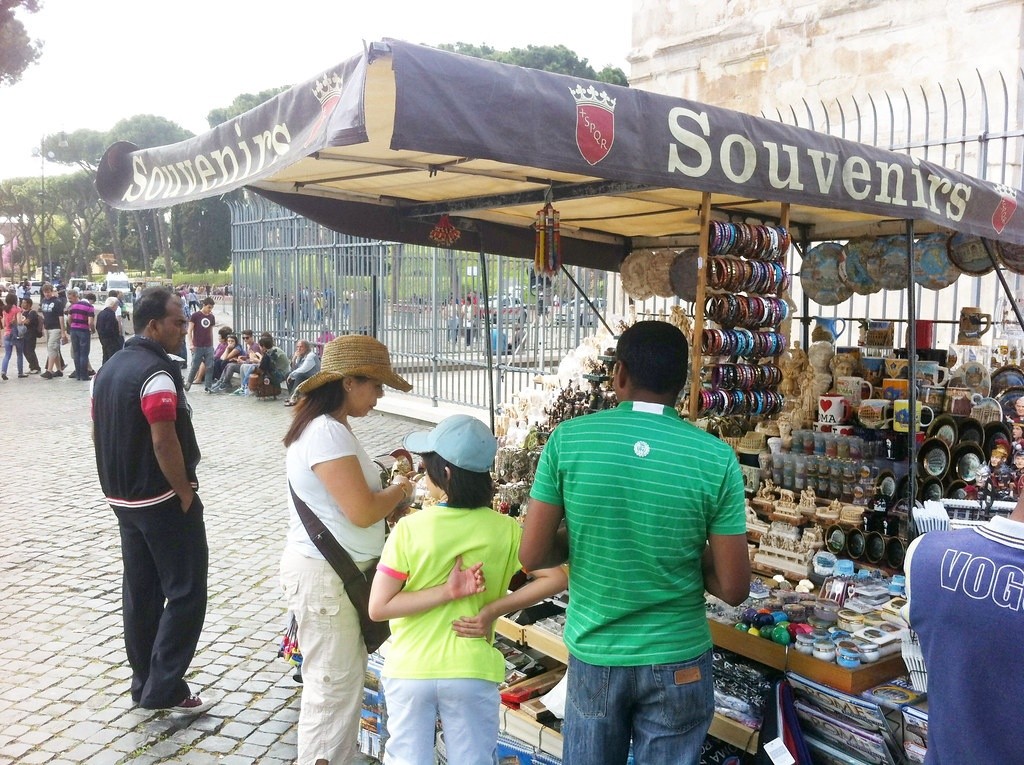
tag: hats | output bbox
[403,413,496,473]
[293,335,414,394]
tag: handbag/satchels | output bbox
[343,560,391,656]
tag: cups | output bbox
[915,320,932,348]
[921,384,946,414]
[884,378,919,400]
[894,399,934,433]
[946,387,972,416]
[813,422,839,434]
[859,400,892,430]
[838,377,873,404]
[868,321,894,346]
[886,359,910,379]
[915,361,949,386]
[862,356,885,386]
[838,348,862,372]
[812,315,846,344]
[818,394,851,423]
[832,426,855,436]
[961,307,991,338]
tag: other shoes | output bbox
[204,388,211,394]
[284,397,296,407]
[77,376,92,381]
[25,369,41,374]
[61,364,68,370]
[185,383,192,391]
[1,372,8,381]
[18,374,29,378]
[89,370,96,376]
[69,371,77,378]
[40,370,63,379]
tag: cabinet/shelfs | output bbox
[495,558,907,765]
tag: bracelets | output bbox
[698,222,788,414]
[391,482,407,498]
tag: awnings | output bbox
[94,38,1024,338]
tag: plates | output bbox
[825,524,845,555]
[865,532,884,564]
[887,537,905,570]
[869,363,1024,510]
[845,528,865,559]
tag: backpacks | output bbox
[29,309,44,339]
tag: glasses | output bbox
[241,337,250,340]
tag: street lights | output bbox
[0,234,6,277]
[31,127,68,314]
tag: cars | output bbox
[475,296,527,324]
[107,273,131,292]
[18,281,52,295]
[553,297,607,327]
[0,277,12,292]
[8,284,20,294]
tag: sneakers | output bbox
[139,693,213,717]
[234,387,245,394]
[211,382,232,393]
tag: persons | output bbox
[801,486,816,507]
[904,488,1024,765]
[520,321,751,765]
[91,286,212,714]
[282,334,413,765]
[184,297,214,393]
[444,292,513,355]
[368,414,567,765]
[746,479,774,522]
[976,397,1024,487]
[174,284,353,404]
[0,277,143,381]
[777,339,856,446]
